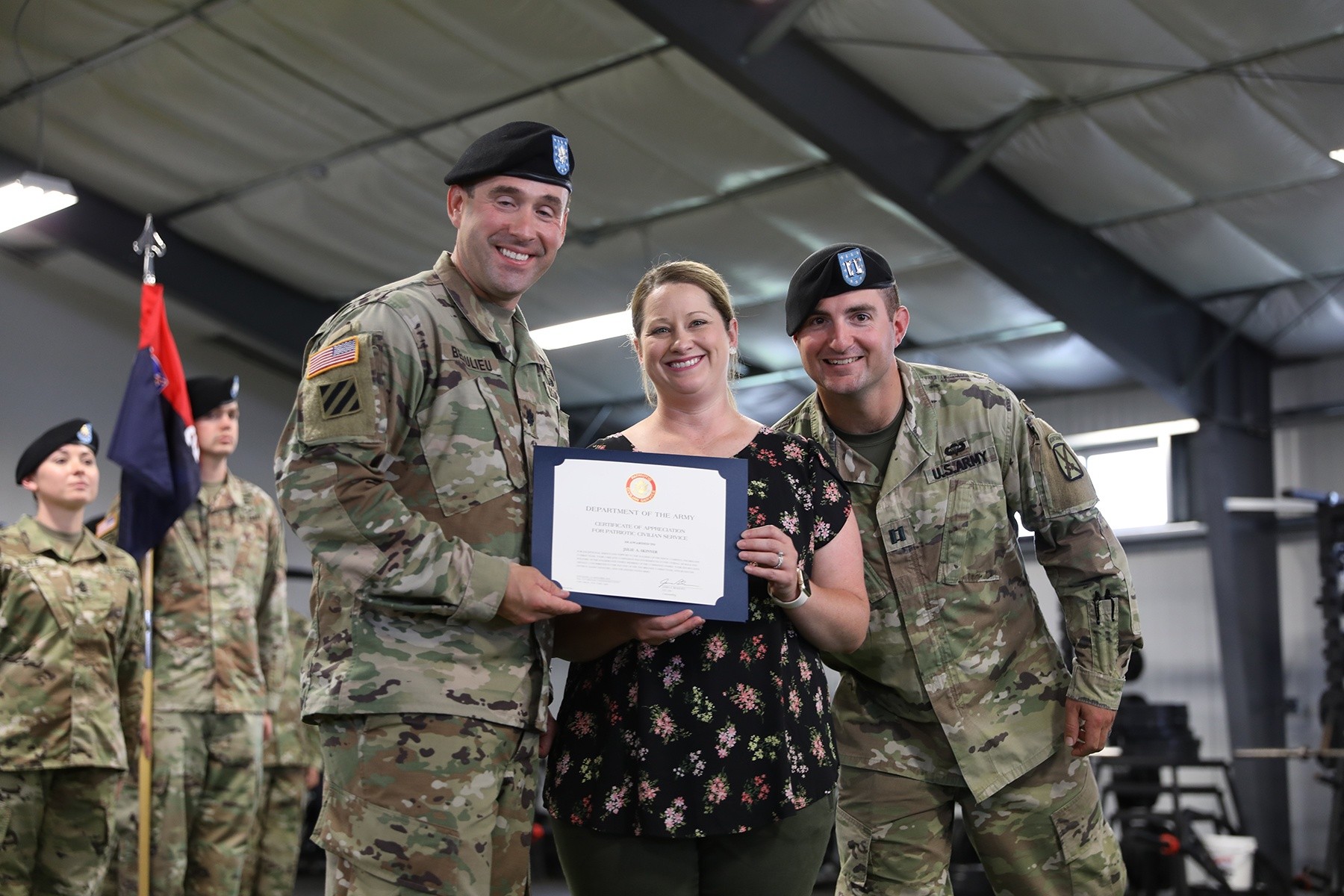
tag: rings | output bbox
[772,553,783,569]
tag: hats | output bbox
[14,417,100,484]
[444,120,577,195]
[183,374,241,422]
[783,243,896,336]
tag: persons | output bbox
[275,122,574,896]
[0,372,324,895]
[760,243,1143,896]
[546,263,869,896]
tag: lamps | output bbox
[0,93,79,232]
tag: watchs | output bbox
[767,568,812,608]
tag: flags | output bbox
[107,285,200,558]
